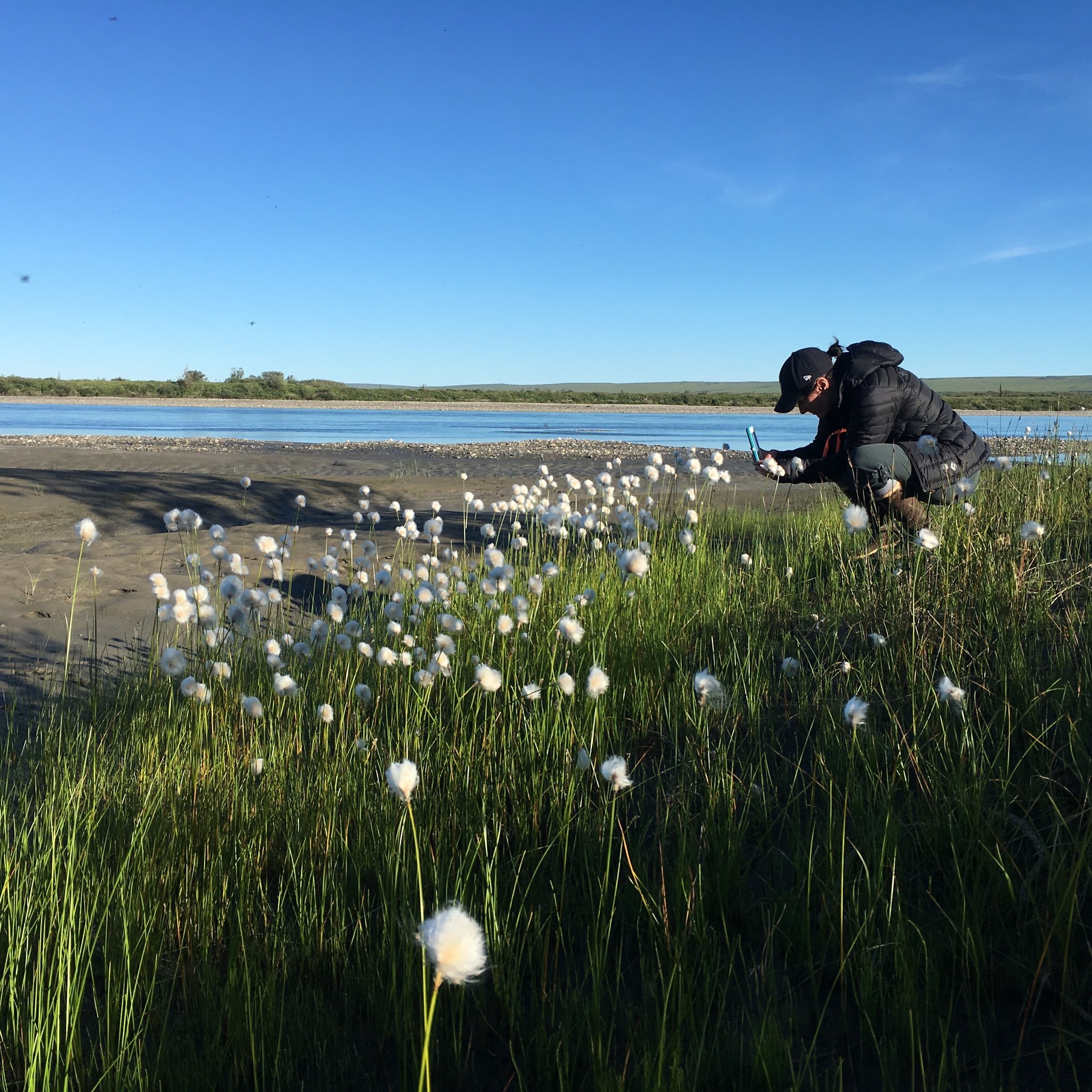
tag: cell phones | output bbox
[746,426,763,462]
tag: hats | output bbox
[773,347,834,414]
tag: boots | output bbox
[839,483,904,559]
[873,480,934,560]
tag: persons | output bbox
[752,341,990,561]
